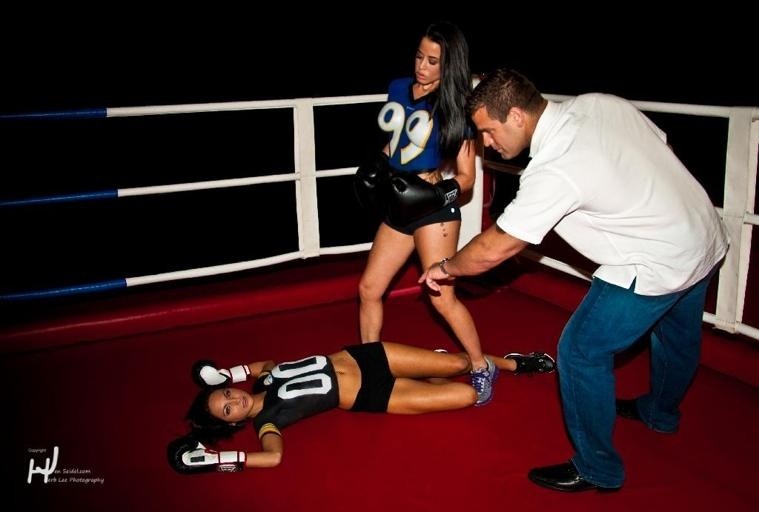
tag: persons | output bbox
[166,340,556,474]
[417,67,732,494]
[353,22,500,407]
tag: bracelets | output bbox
[439,257,450,276]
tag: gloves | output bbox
[192,360,251,390]
[167,436,247,474]
[352,151,460,227]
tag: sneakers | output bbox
[504,351,556,375]
[470,354,499,407]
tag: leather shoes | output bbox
[528,460,620,491]
[616,397,642,420]
